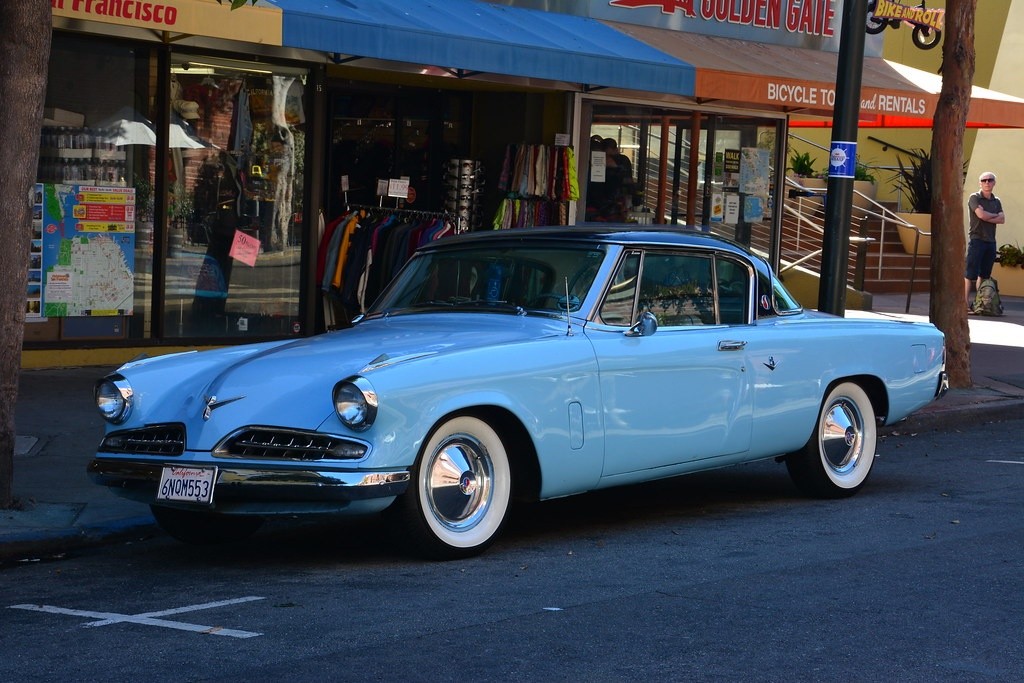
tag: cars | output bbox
[91,223,949,562]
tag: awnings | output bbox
[51,0,1024,127]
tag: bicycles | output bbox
[865,0,942,50]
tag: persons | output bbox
[586,138,633,218]
[964,172,1005,315]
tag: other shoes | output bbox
[966,306,975,315]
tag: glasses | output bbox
[441,160,485,236]
[981,178,996,183]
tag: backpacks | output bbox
[972,276,1004,317]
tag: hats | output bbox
[174,101,200,119]
